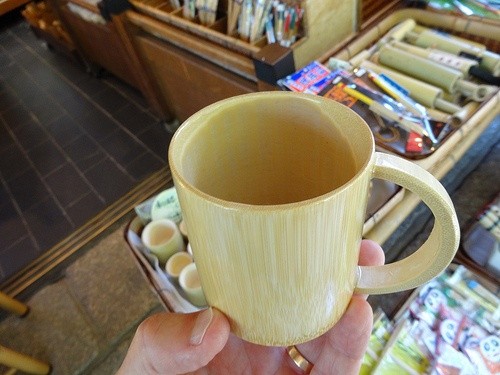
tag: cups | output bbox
[178,264,209,307]
[186,241,193,254]
[165,252,193,283]
[179,220,188,237]
[167,91,460,347]
[140,219,186,267]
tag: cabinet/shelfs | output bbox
[31,0,407,134]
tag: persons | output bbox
[113,238,386,375]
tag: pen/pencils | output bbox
[264,2,303,46]
[338,73,432,137]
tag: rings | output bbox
[284,345,315,375]
